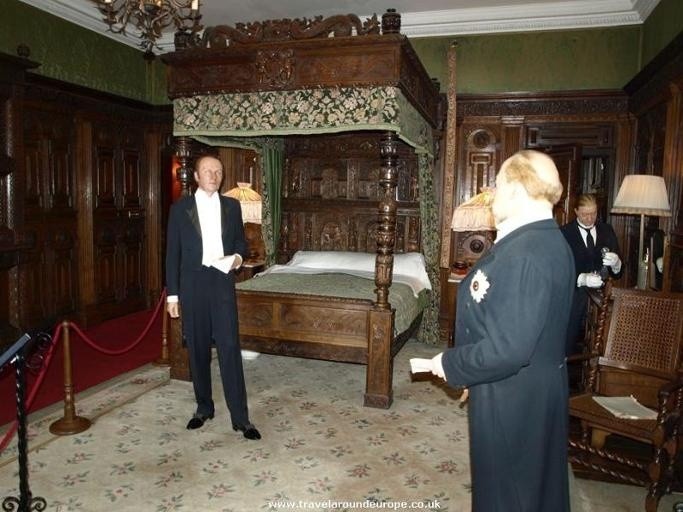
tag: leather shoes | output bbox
[233,420,261,440]
[187,409,214,429]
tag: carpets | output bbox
[1,330,683,512]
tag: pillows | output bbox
[288,249,429,278]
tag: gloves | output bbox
[577,273,603,289]
[602,252,622,274]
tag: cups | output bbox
[601,247,610,257]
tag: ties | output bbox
[577,223,596,249]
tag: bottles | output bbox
[637,247,655,291]
[381,8,399,35]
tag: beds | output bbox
[168,251,435,410]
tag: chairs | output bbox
[565,287,682,512]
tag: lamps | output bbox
[94,0,203,58]
[610,174,672,291]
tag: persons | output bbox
[557,189,623,363]
[426,147,579,511]
[163,154,265,441]
